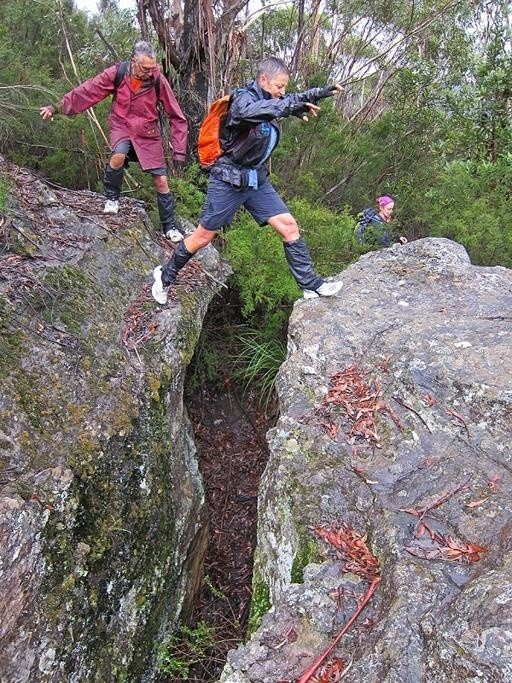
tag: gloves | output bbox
[293,103,311,120]
[323,83,335,97]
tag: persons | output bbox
[151,57,342,305]
[353,197,407,256]
[39,39,188,243]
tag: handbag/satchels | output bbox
[353,207,381,238]
[197,87,254,168]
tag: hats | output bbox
[379,195,394,207]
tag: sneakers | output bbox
[303,281,343,299]
[166,229,184,242]
[152,265,172,304]
[104,200,120,214]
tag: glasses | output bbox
[139,63,159,74]
